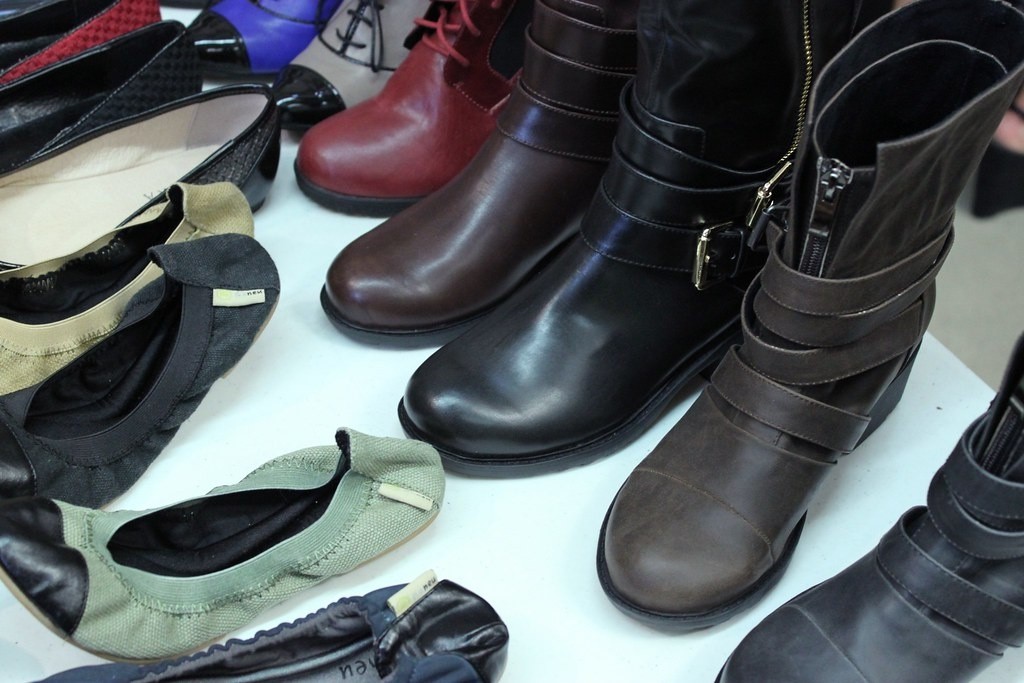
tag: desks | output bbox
[2,8,1023,683]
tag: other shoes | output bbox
[2,1,283,509]
[34,577,507,681]
[0,428,446,662]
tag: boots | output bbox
[715,334,1024,683]
[596,1,1022,637]
[190,0,801,477]
